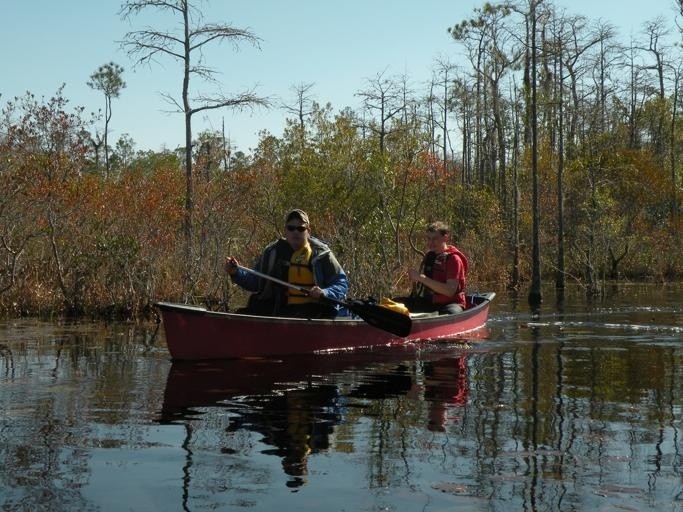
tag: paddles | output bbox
[228,260,412,338]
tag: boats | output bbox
[152,292,497,361]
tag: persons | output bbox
[225,208,348,319]
[395,220,468,315]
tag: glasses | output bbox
[286,226,310,232]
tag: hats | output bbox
[285,209,309,224]
